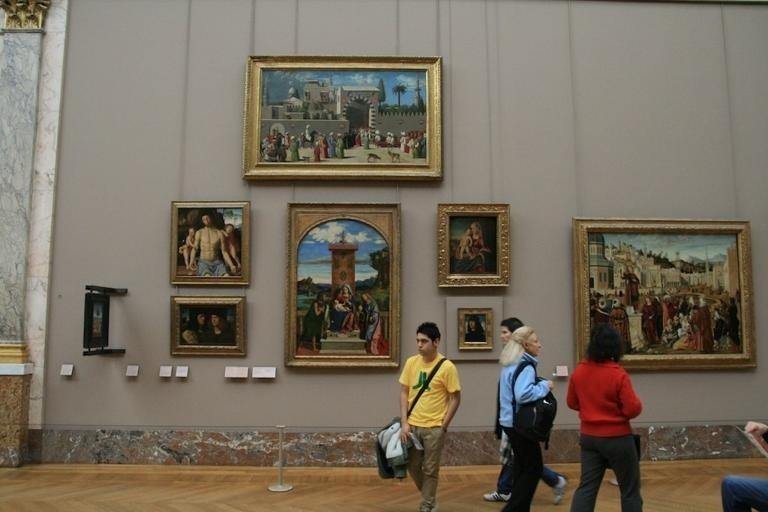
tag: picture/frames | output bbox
[168,294,246,358]
[436,202,510,289]
[456,306,494,350]
[243,54,443,183]
[168,201,253,288]
[570,216,758,371]
[283,201,402,368]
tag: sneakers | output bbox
[484,492,510,502]
[552,475,567,505]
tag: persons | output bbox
[464,315,486,342]
[498,324,555,511]
[260,120,428,164]
[396,320,462,511]
[179,305,236,345]
[458,228,472,259]
[298,281,382,354]
[720,419,768,512]
[478,316,569,507]
[469,221,491,265]
[179,213,241,277]
[588,276,742,353]
[561,326,648,511]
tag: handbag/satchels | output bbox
[376,417,404,479]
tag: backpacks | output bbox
[512,362,557,441]
